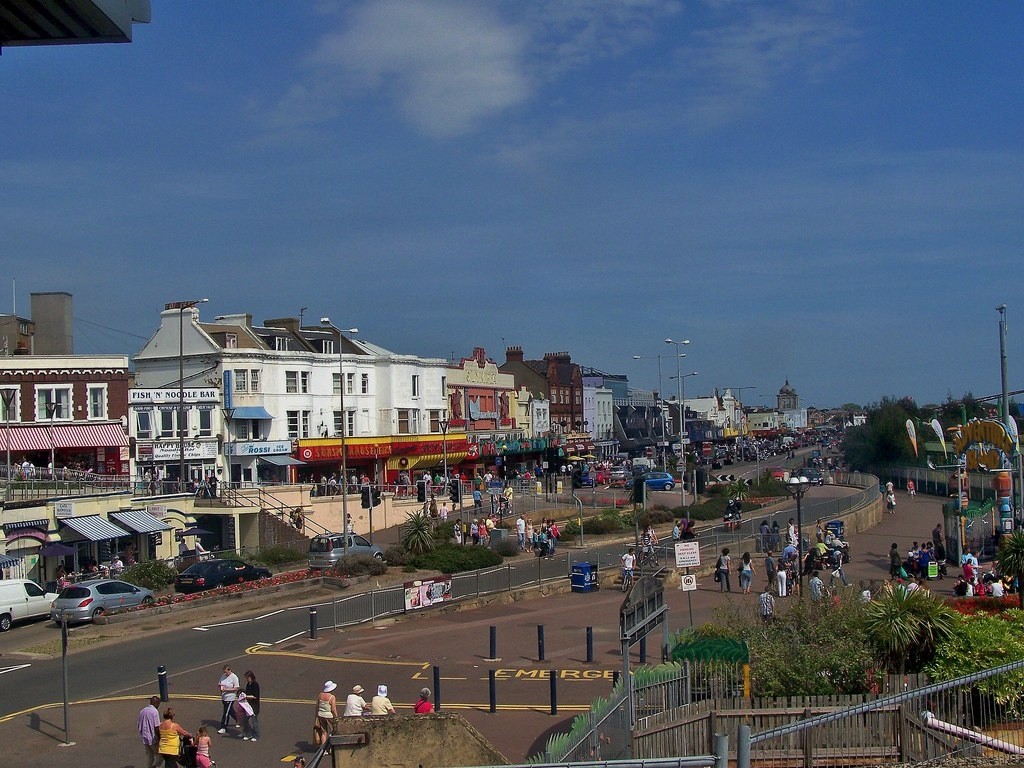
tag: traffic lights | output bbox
[447,478,460,502]
[371,487,381,507]
[416,480,426,502]
[360,485,371,510]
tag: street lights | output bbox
[669,371,698,455]
[664,338,691,507]
[783,468,811,607]
[320,316,360,559]
[43,402,59,480]
[0,387,17,501]
[633,354,688,473]
[437,419,449,496]
[178,298,211,497]
[723,386,757,464]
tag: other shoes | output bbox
[243,735,253,740]
[251,737,258,742]
[747,590,751,594]
[235,724,240,728]
[744,592,747,595]
[218,728,226,733]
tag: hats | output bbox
[378,685,387,697]
[323,681,338,692]
[828,530,833,534]
[353,685,364,694]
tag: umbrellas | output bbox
[177,528,215,550]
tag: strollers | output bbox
[175,732,219,768]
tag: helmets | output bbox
[728,499,734,506]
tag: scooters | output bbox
[723,510,742,533]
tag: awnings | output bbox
[106,507,176,535]
[56,513,131,542]
[258,454,308,466]
[230,405,273,420]
[0,423,130,450]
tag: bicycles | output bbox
[638,544,658,568]
[620,565,636,592]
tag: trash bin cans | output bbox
[827,520,845,538]
[491,529,508,549]
[572,562,598,591]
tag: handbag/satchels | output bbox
[714,571,721,582]
[831,569,840,578]
[892,500,896,506]
[313,727,320,746]
[737,560,744,571]
[900,567,907,578]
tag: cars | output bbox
[624,471,675,491]
[796,467,825,488]
[592,438,853,488]
[51,579,157,627]
[173,559,273,594]
[0,578,61,633]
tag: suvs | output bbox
[308,531,384,575]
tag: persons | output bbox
[739,552,756,595]
[886,491,896,513]
[809,570,824,603]
[620,548,636,588]
[907,479,916,499]
[757,586,775,623]
[294,680,434,768]
[217,664,261,742]
[136,695,211,768]
[299,427,1024,606]
[717,548,732,592]
[13,457,220,585]
[932,523,946,559]
